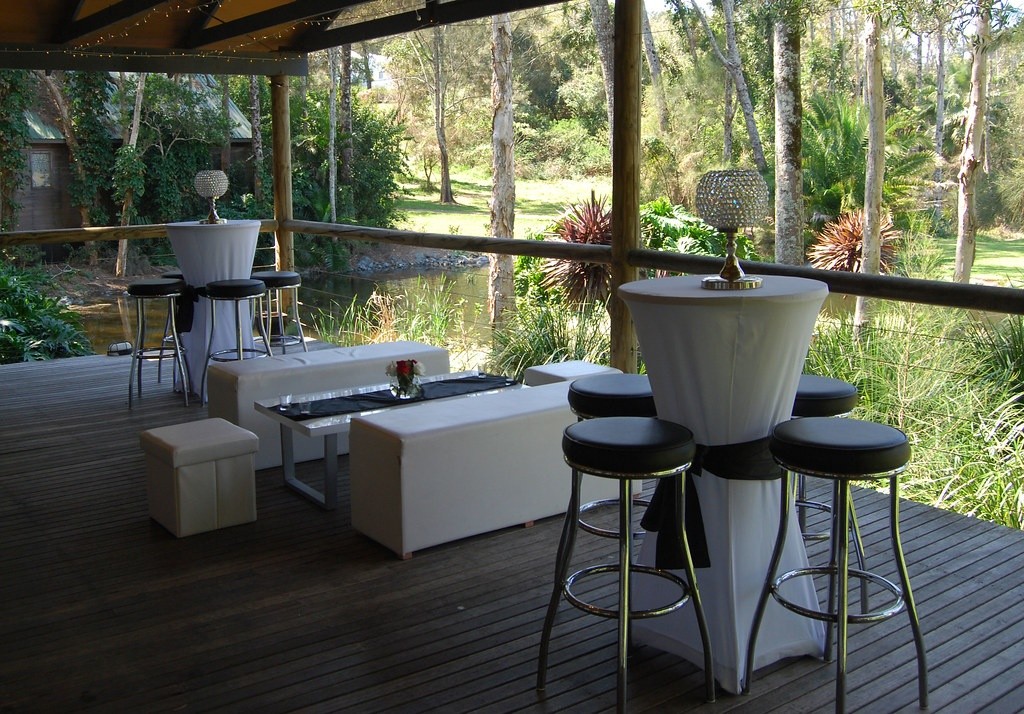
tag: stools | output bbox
[157,272,187,384]
[527,360,622,388]
[554,373,658,607]
[743,417,931,714]
[250,270,308,356]
[126,278,192,410]
[790,372,869,615]
[200,279,273,407]
[536,417,714,714]
[140,417,259,537]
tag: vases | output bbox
[390,377,422,399]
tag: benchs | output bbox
[349,379,642,562]
[205,340,450,471]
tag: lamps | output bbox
[195,169,229,225]
[694,169,769,291]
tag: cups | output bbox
[299,394,310,413]
[279,392,293,411]
[478,364,486,378]
[505,371,513,384]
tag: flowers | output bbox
[384,359,425,380]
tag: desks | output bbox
[253,371,530,509]
[620,274,830,695]
[165,220,261,401]
